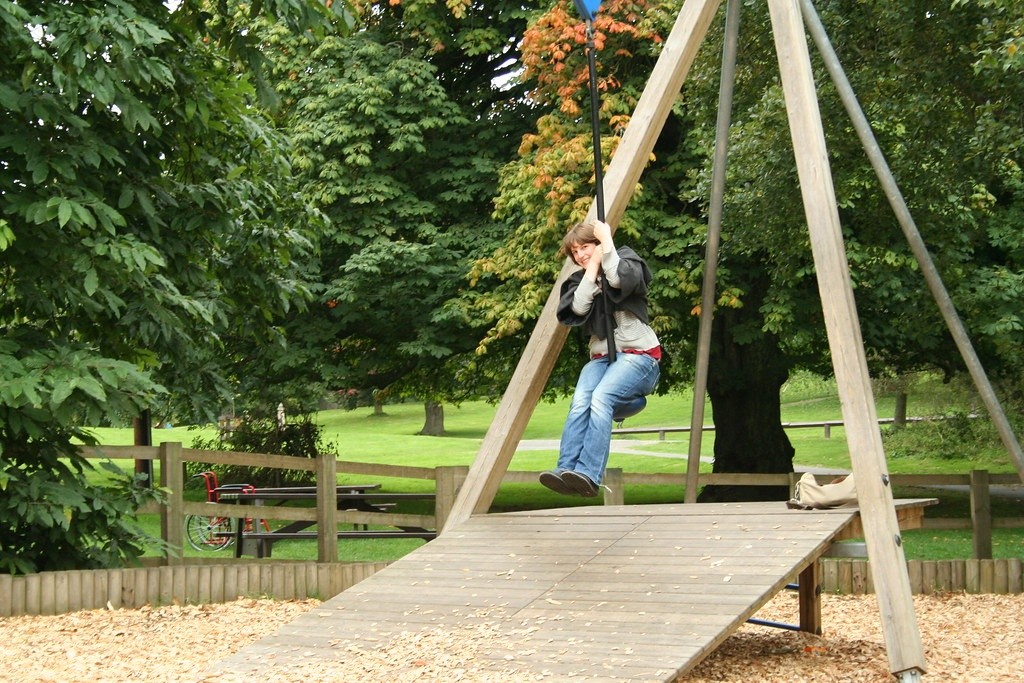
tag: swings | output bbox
[569,0,649,425]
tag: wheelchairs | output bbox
[184,470,272,553]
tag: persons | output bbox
[540,219,661,497]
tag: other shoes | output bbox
[539,471,577,495]
[560,470,599,497]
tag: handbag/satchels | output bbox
[785,471,860,510]
